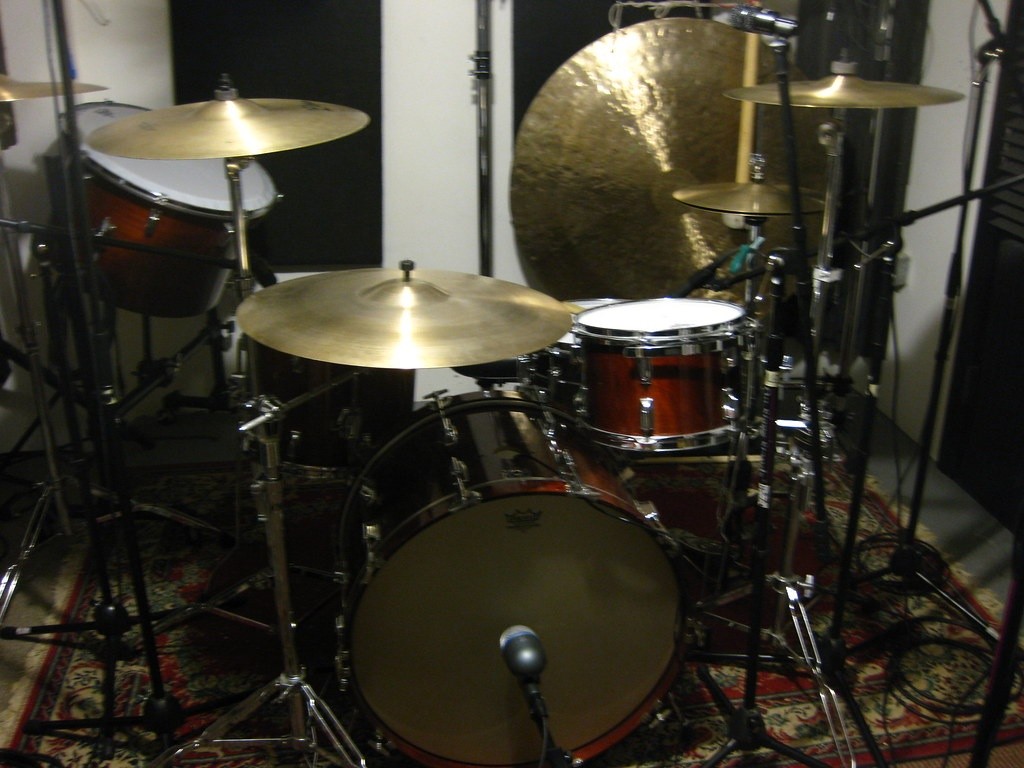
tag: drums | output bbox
[248,274,416,476]
[568,296,752,457]
[336,388,697,761]
[49,101,285,319]
[541,296,635,429]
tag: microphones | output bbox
[670,251,732,298]
[979,0,1011,61]
[248,252,276,288]
[499,625,552,722]
[729,5,800,34]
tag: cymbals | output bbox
[0,72,111,113]
[81,96,373,165]
[670,175,831,219]
[238,254,571,374]
[509,15,832,301]
[717,72,971,115]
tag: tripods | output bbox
[0,1,371,768]
[655,35,1022,768]
